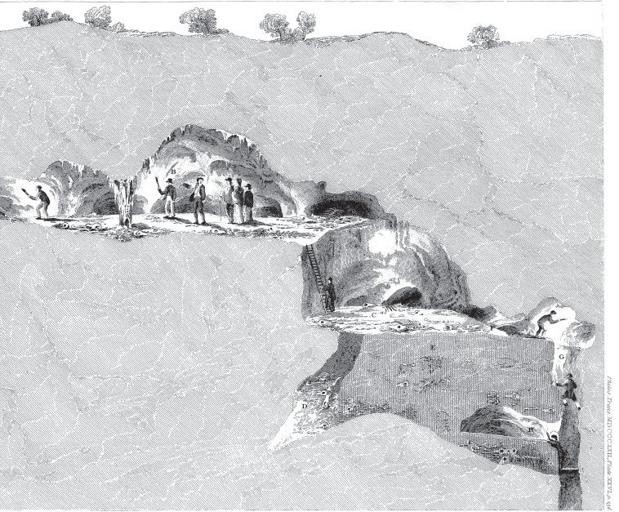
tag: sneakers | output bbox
[202,222,206,224]
[163,216,175,219]
[227,221,254,224]
[564,403,568,406]
[193,222,198,224]
[578,407,581,410]
[36,217,42,219]
[43,218,49,221]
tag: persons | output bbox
[536,310,560,337]
[556,372,582,411]
[547,435,566,469]
[321,277,336,312]
[231,178,244,223]
[156,178,177,218]
[221,178,235,224]
[27,185,52,221]
[242,184,253,224]
[189,178,207,224]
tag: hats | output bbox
[245,184,251,187]
[225,178,231,181]
[237,178,242,182]
[166,178,172,182]
[196,177,204,182]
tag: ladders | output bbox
[306,244,334,316]
